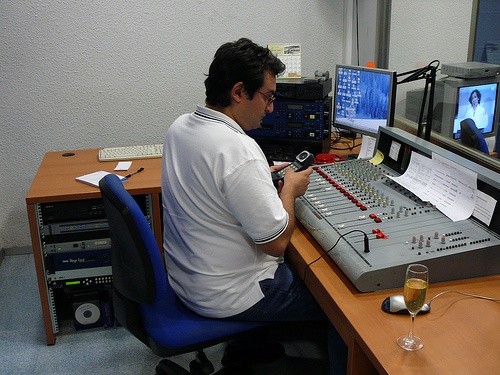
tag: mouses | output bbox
[381,294,430,313]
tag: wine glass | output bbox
[397,264,428,351]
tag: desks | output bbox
[26,146,164,345]
[279,129,500,375]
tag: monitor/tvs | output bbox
[333,65,397,137]
[405,76,500,142]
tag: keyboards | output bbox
[98,143,164,161]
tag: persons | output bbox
[161,38,348,375]
[465,90,485,132]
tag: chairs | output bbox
[96,174,259,375]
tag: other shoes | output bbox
[221,342,285,370]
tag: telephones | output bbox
[271,148,315,185]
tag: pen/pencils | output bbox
[120,167,145,180]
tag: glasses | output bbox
[246,84,276,105]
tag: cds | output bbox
[75,303,100,325]
